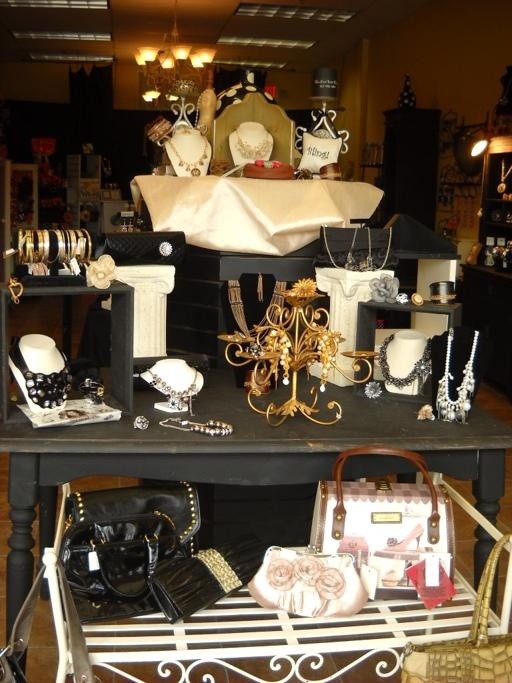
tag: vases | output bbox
[398,72,416,106]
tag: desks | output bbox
[1,354,511,682]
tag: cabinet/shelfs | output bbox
[101,199,130,232]
[66,153,103,234]
[458,153,511,302]
[2,276,134,425]
[353,298,462,404]
[1,159,39,283]
[378,105,436,236]
[42,472,511,682]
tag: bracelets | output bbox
[16,226,92,266]
[78,377,106,398]
[431,293,456,301]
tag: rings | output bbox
[133,415,151,430]
[396,293,407,304]
[412,293,424,306]
[418,404,433,423]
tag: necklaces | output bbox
[146,366,201,411]
[377,333,432,388]
[169,136,212,177]
[345,227,376,272]
[235,128,273,162]
[10,332,77,414]
[159,416,234,436]
[217,272,293,353]
[436,327,479,424]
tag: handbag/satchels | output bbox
[247,546,369,620]
[313,226,399,270]
[149,533,267,625]
[57,480,202,554]
[400,533,512,683]
[95,232,187,266]
[309,446,457,600]
[56,512,189,625]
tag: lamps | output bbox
[487,133,512,184]
[142,78,179,104]
[170,79,202,128]
[294,66,349,153]
[454,111,489,159]
[132,0,216,70]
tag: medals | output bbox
[497,183,506,193]
